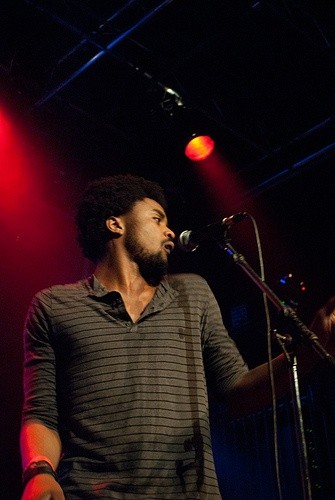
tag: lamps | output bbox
[170,105,215,162]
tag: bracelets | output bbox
[22,456,58,487]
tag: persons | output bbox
[19,173,335,500]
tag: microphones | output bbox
[178,211,248,252]
